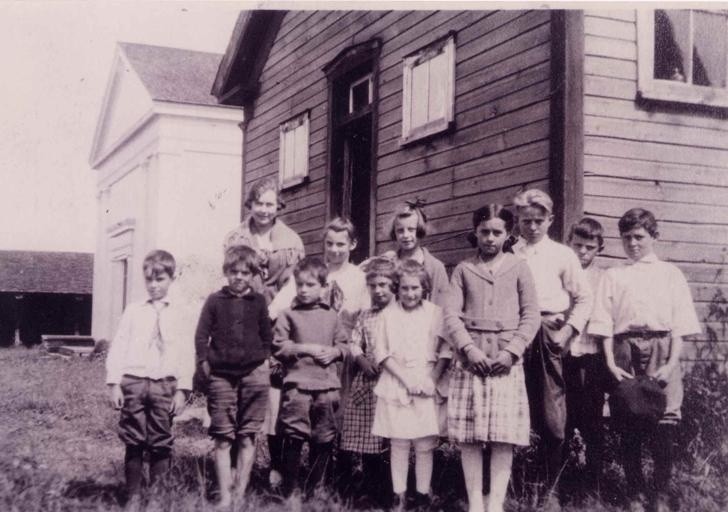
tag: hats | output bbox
[610,374,666,435]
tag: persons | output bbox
[104,251,195,512]
[196,177,703,511]
